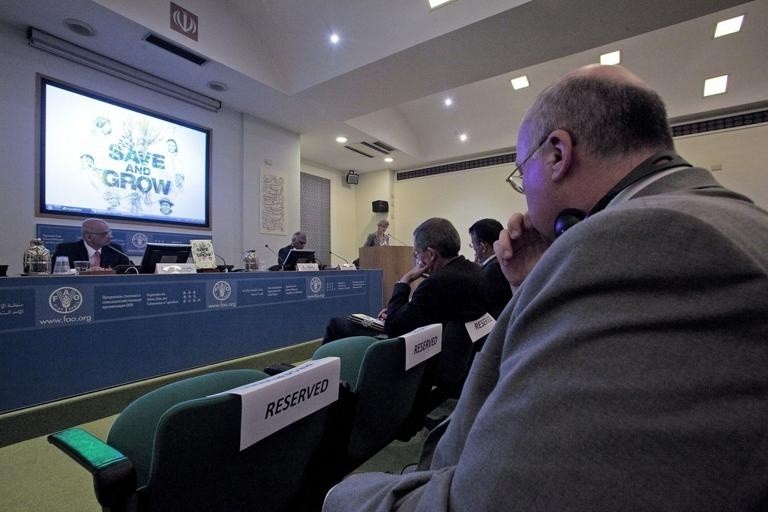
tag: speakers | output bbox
[372,200,389,213]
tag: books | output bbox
[348,312,386,333]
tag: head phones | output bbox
[552,147,694,236]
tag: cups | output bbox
[73,260,90,275]
[51,255,72,275]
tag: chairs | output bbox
[49,309,494,510]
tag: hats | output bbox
[159,197,174,205]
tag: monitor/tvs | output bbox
[140,243,192,274]
[279,249,314,271]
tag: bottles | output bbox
[244,248,260,273]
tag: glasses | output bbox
[89,229,112,237]
[505,134,555,193]
[412,247,426,258]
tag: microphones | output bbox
[105,243,142,272]
[264,244,287,268]
[329,251,357,269]
[387,234,407,247]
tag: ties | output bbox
[94,252,101,266]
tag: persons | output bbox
[468,218,513,322]
[172,171,185,191]
[363,218,392,247]
[165,137,179,154]
[322,58,768,511]
[157,197,175,215]
[95,115,112,135]
[277,231,308,266]
[79,153,95,170]
[321,217,486,392]
[50,217,137,273]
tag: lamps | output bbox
[347,171,358,185]
